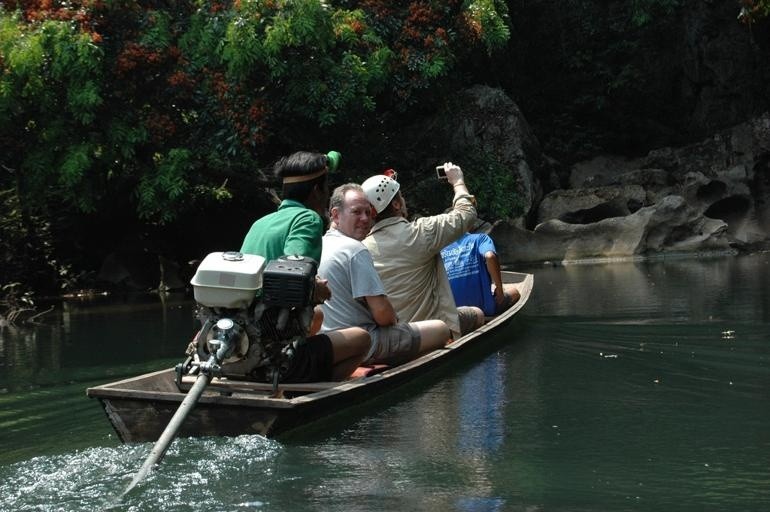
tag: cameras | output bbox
[435,166,448,180]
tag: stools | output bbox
[177,372,348,396]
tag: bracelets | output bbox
[451,182,465,187]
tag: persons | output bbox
[360,161,484,341]
[311,182,451,370]
[438,206,522,316]
[238,150,373,382]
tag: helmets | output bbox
[361,175,400,218]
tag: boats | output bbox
[86,251,535,497]
